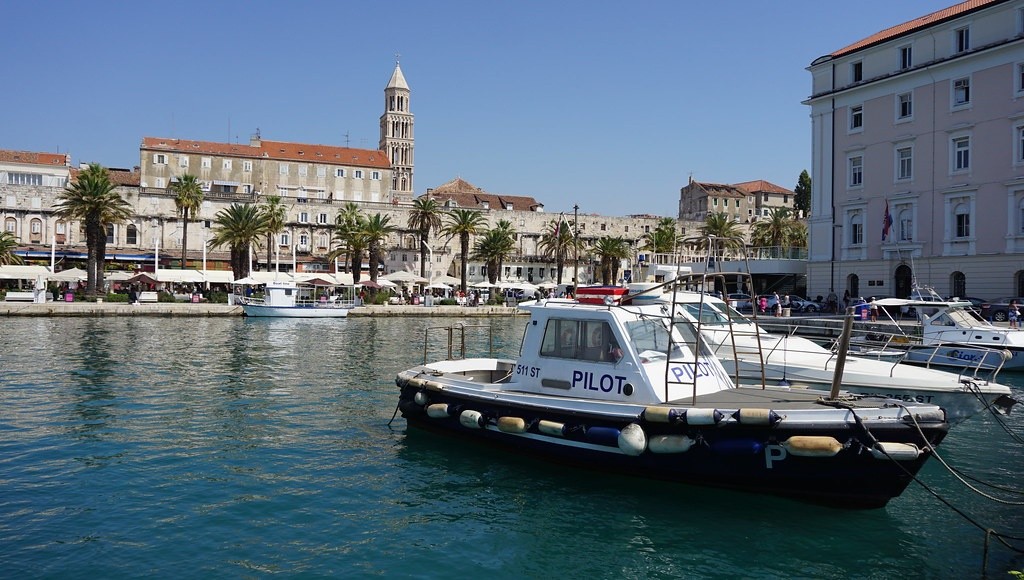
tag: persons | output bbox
[1,259,573,309]
[690,287,911,324]
[1008,299,1020,329]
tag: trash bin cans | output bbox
[854,303,871,321]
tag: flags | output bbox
[881,202,893,242]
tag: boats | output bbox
[641,291,1013,429]
[837,297,1024,375]
[394,287,951,519]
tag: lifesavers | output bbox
[592,326,602,346]
[561,328,575,346]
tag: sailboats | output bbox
[239,280,355,317]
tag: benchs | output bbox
[5,292,54,304]
[139,292,158,302]
